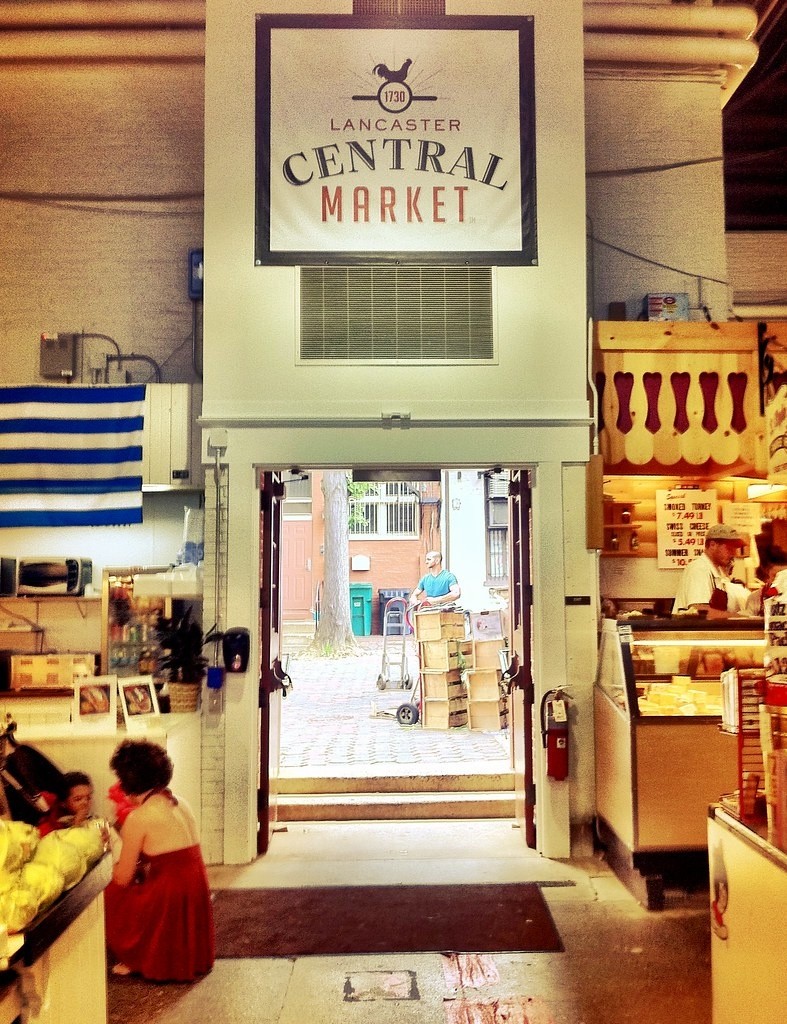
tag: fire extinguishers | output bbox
[540,684,574,781]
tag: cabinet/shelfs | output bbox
[603,501,644,556]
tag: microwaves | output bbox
[16,555,92,595]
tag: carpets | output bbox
[210,882,565,958]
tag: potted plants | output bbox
[152,604,217,713]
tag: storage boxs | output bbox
[414,610,510,730]
[0,628,46,653]
[10,653,95,691]
[643,293,689,321]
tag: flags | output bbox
[0,385,147,528]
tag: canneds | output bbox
[111,623,149,666]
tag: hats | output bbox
[706,524,747,548]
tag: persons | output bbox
[746,551,787,615]
[58,771,93,827]
[411,550,461,604]
[104,741,216,982]
[672,525,748,618]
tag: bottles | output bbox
[112,646,157,677]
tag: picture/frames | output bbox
[74,674,117,734]
[118,675,160,723]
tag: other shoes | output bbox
[111,962,130,976]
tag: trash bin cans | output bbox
[349,582,373,636]
[378,588,410,636]
